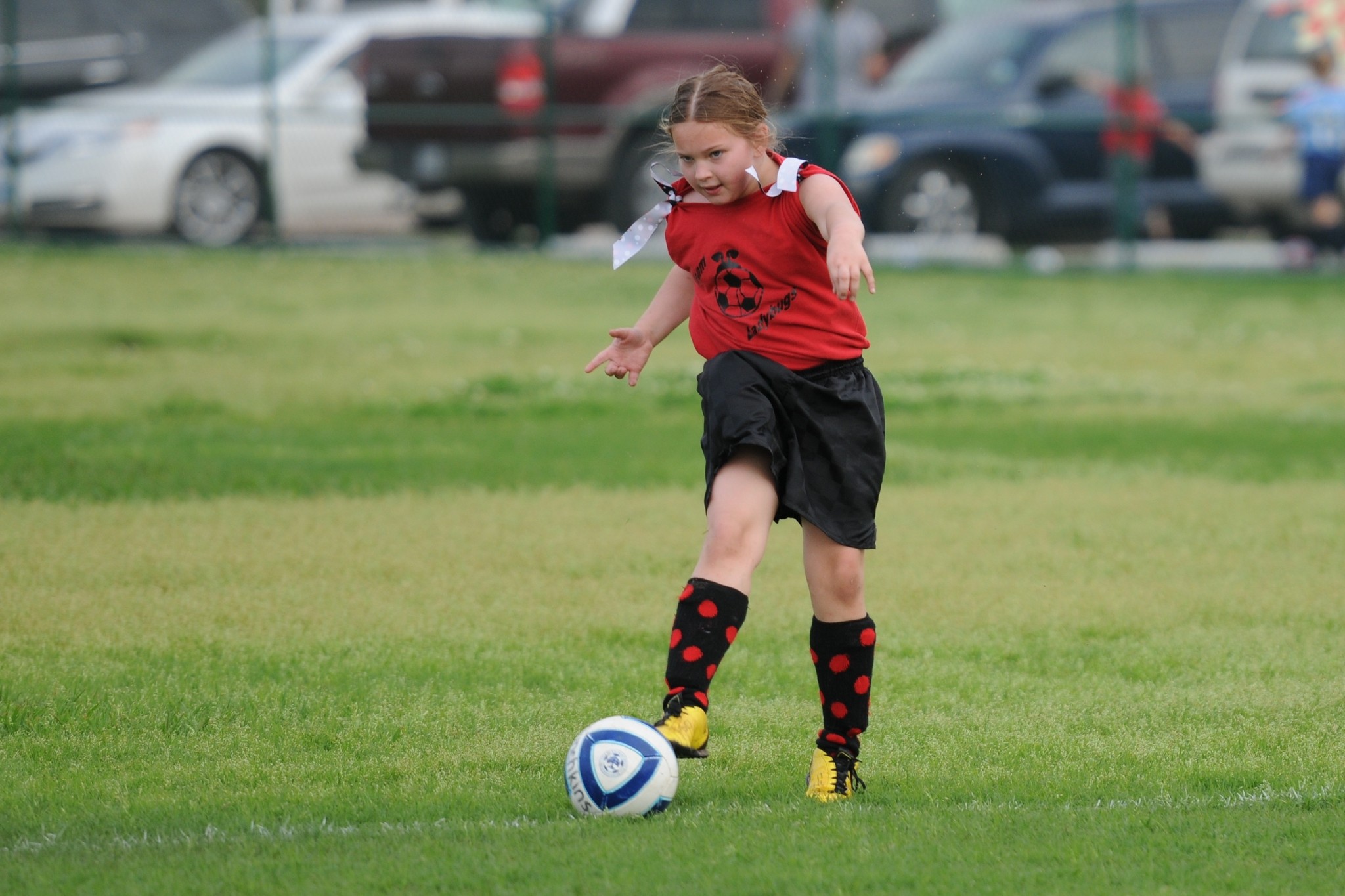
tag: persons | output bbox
[584,65,887,803]
[1078,67,1208,272]
[1268,39,1345,277]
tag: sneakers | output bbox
[803,746,860,805]
[648,705,710,758]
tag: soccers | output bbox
[564,716,680,820]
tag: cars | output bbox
[2,5,554,251]
[750,1,1345,248]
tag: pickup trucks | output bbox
[346,1,802,251]
